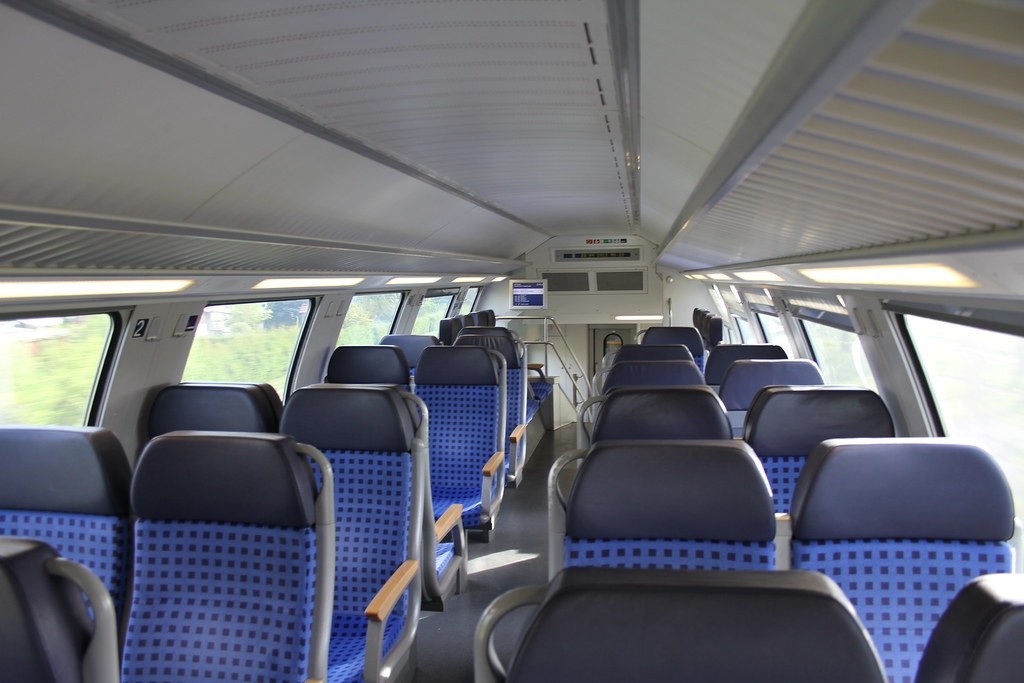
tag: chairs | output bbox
[279,386,423,683]
[414,345,506,542]
[439,309,554,425]
[278,383,470,611]
[601,344,695,390]
[379,334,441,393]
[915,574,1024,683]
[593,361,707,411]
[635,326,704,373]
[789,437,1016,683]
[547,438,775,582]
[692,307,723,353]
[0,426,133,633]
[705,344,789,396]
[0,534,120,682]
[451,333,527,488]
[120,428,337,683]
[143,384,278,438]
[474,567,888,683]
[719,359,826,438]
[178,381,284,428]
[324,345,409,391]
[743,384,896,513]
[577,384,732,468]
